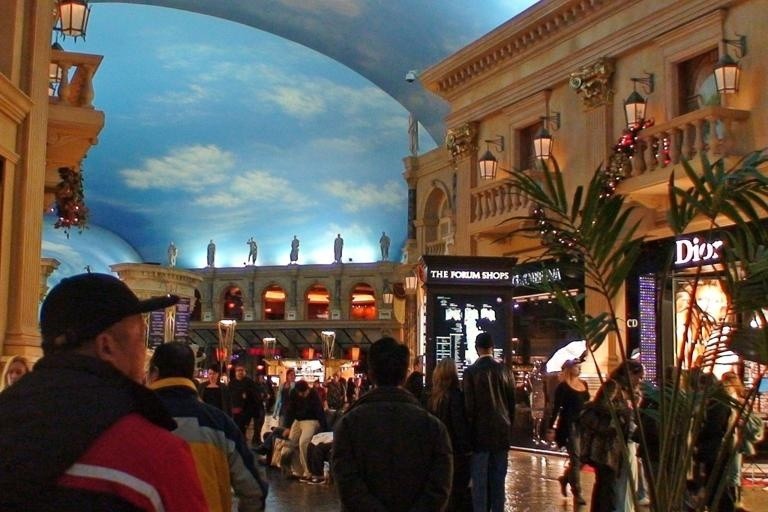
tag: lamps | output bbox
[381,278,394,303]
[531,110,561,161]
[48,57,63,99]
[713,29,747,95]
[621,70,656,133]
[478,134,505,182]
[51,2,93,41]
[402,269,418,295]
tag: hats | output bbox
[476,333,493,345]
[41,273,180,348]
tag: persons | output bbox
[195,365,372,486]
[378,232,391,260]
[330,335,454,512]
[145,341,269,511]
[421,358,473,512]
[523,360,545,445]
[290,236,299,264]
[333,233,343,261]
[407,354,422,397]
[0,354,30,391]
[542,356,745,512]
[0,273,208,511]
[466,333,516,508]
[205,240,215,267]
[168,241,178,269]
[247,236,258,264]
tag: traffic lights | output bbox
[216,348,227,361]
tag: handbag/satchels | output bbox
[578,404,625,442]
[271,437,288,468]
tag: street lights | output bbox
[262,337,278,384]
[218,319,238,381]
[319,331,336,382]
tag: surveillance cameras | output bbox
[405,71,416,83]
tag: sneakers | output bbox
[300,476,326,485]
[574,496,585,505]
[636,498,650,506]
[557,476,567,496]
